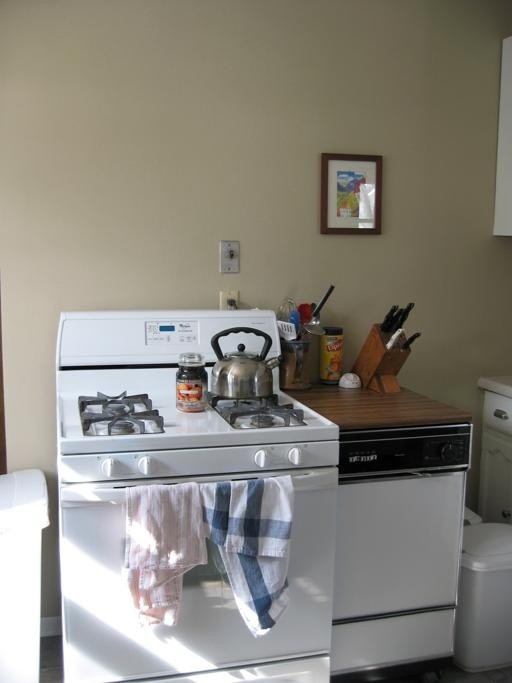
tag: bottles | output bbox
[319,326,344,384]
[176,352,209,412]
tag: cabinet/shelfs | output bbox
[476,375,512,525]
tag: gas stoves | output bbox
[55,309,339,484]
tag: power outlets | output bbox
[218,289,238,310]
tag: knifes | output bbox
[394,303,414,335]
[390,308,405,334]
[402,333,421,351]
[386,328,405,351]
[380,304,399,332]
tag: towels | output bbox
[123,481,208,630]
[198,474,295,640]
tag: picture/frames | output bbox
[320,152,383,235]
